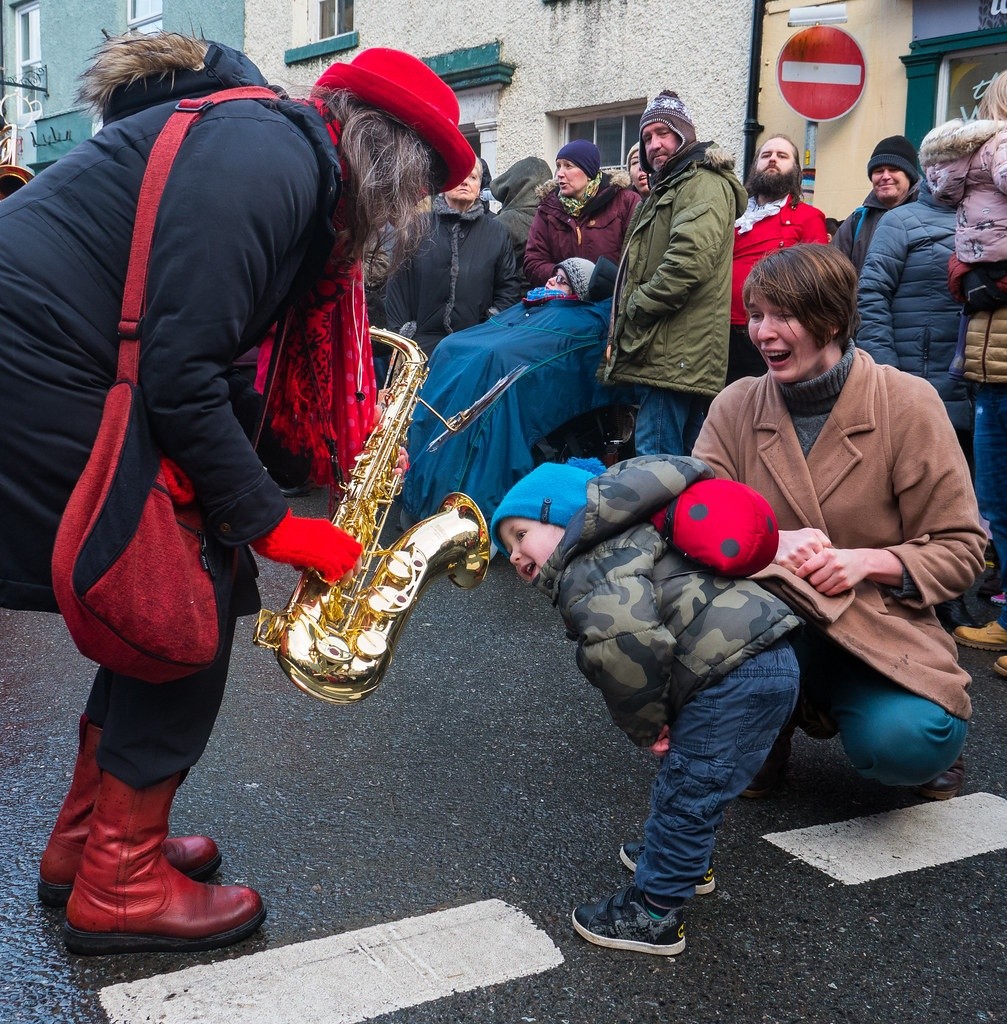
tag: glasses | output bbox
[553,271,571,287]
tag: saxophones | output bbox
[253,320,490,705]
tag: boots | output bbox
[62,769,266,956]
[978,540,1001,595]
[38,711,221,906]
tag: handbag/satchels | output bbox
[53,377,234,686]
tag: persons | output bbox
[690,244,988,802]
[224,73,1006,677]
[490,453,801,955]
[0,46,476,948]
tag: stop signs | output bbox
[775,23,867,122]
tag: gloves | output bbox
[960,263,1007,316]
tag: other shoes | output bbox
[741,760,786,799]
[920,751,965,800]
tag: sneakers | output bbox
[934,593,983,627]
[619,840,715,894]
[571,886,685,956]
[952,620,1007,651]
[990,592,1007,604]
[993,656,1007,678]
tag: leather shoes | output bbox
[278,480,314,498]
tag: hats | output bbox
[868,135,918,184]
[626,141,639,173]
[489,457,606,559]
[639,90,696,174]
[556,139,600,180]
[310,48,475,192]
[552,257,596,302]
